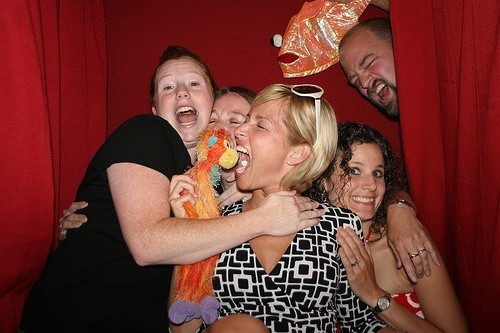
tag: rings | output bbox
[410,253,419,258]
[418,249,426,253]
[351,262,359,266]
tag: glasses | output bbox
[289,84,323,148]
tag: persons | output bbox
[13,45,471,333]
[338,18,401,118]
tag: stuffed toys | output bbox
[167,127,239,325]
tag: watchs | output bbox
[372,291,391,315]
[390,199,416,211]
